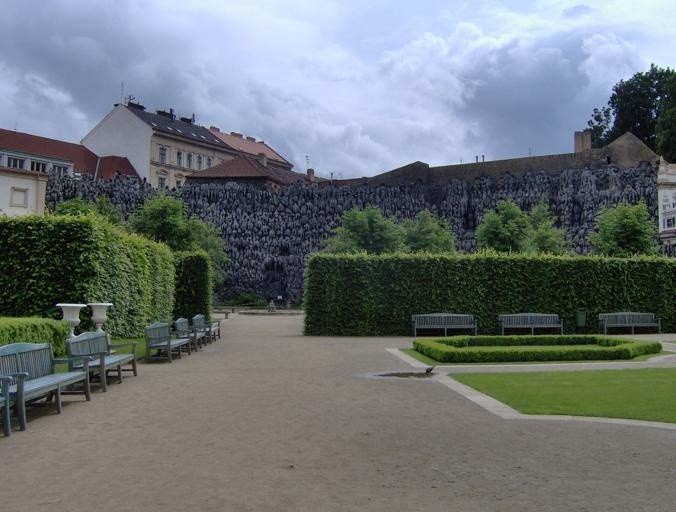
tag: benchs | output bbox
[411,313,478,337]
[0,376,13,435]
[598,313,662,335]
[499,313,564,335]
[142,316,221,363]
[0,343,94,432]
[66,332,137,393]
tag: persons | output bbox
[268,299,277,312]
